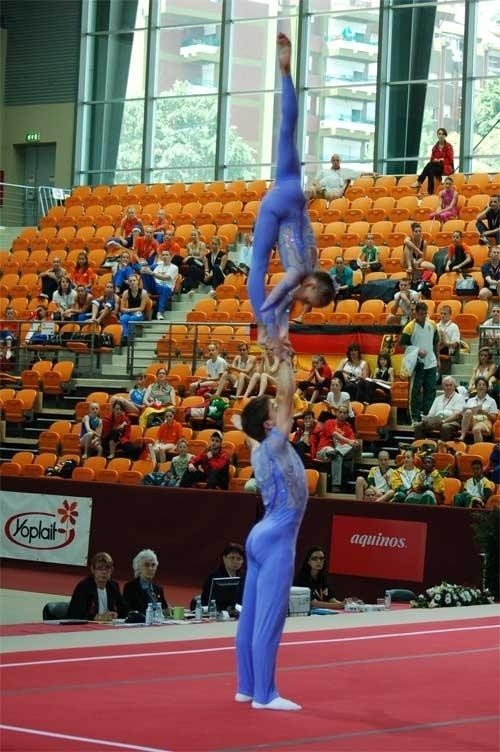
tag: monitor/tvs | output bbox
[208,576,244,620]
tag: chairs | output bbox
[42,602,71,623]
[389,589,417,601]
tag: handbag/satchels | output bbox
[454,278,478,296]
[30,332,114,348]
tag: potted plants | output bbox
[470,511,500,593]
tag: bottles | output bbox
[384,591,392,610]
[146,598,221,625]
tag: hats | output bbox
[211,430,223,439]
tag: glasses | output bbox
[311,556,324,561]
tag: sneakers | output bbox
[156,312,164,320]
[408,180,421,188]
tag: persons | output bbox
[327,177,500,323]
[311,151,356,203]
[245,31,337,362]
[411,127,454,197]
[232,338,315,714]
[60,548,129,623]
[223,223,255,271]
[199,544,244,615]
[120,548,169,616]
[0,206,226,369]
[292,545,350,612]
[44,302,499,512]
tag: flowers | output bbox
[409,575,493,608]
